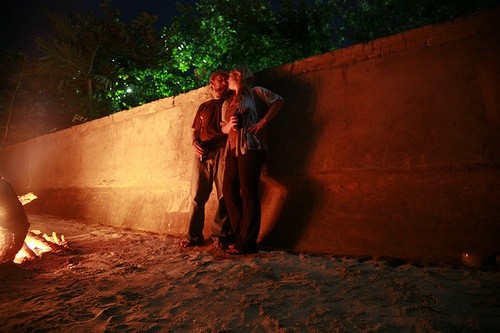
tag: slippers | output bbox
[225,247,259,255]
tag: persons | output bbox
[221,68,284,255]
[183,71,226,246]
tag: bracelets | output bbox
[263,118,267,124]
[191,139,197,145]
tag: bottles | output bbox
[199,142,208,162]
[233,109,243,130]
